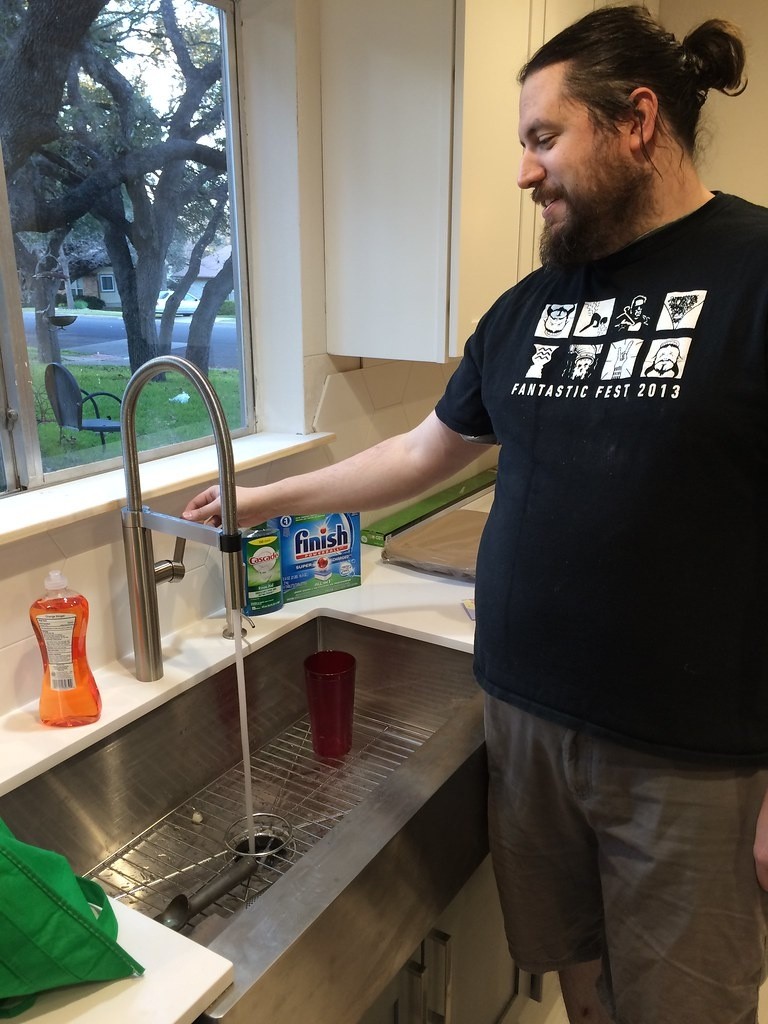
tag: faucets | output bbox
[118,353,245,686]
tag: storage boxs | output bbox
[360,465,498,547]
[267,512,360,603]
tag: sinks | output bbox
[0,595,490,954]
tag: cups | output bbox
[304,649,357,758]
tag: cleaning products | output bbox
[240,519,285,615]
[29,566,101,728]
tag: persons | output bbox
[181,6,768,1024]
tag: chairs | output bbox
[45,363,122,454]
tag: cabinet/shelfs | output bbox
[319,0,660,364]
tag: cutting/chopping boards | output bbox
[380,510,490,581]
